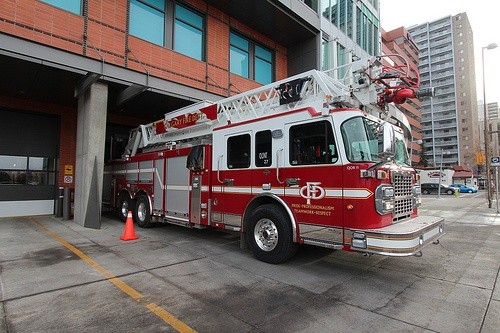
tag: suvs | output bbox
[420,183,455,195]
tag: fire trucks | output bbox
[108,53,447,266]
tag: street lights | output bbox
[481,43,499,208]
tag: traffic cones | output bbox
[119,210,140,241]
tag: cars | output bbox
[448,184,477,194]
